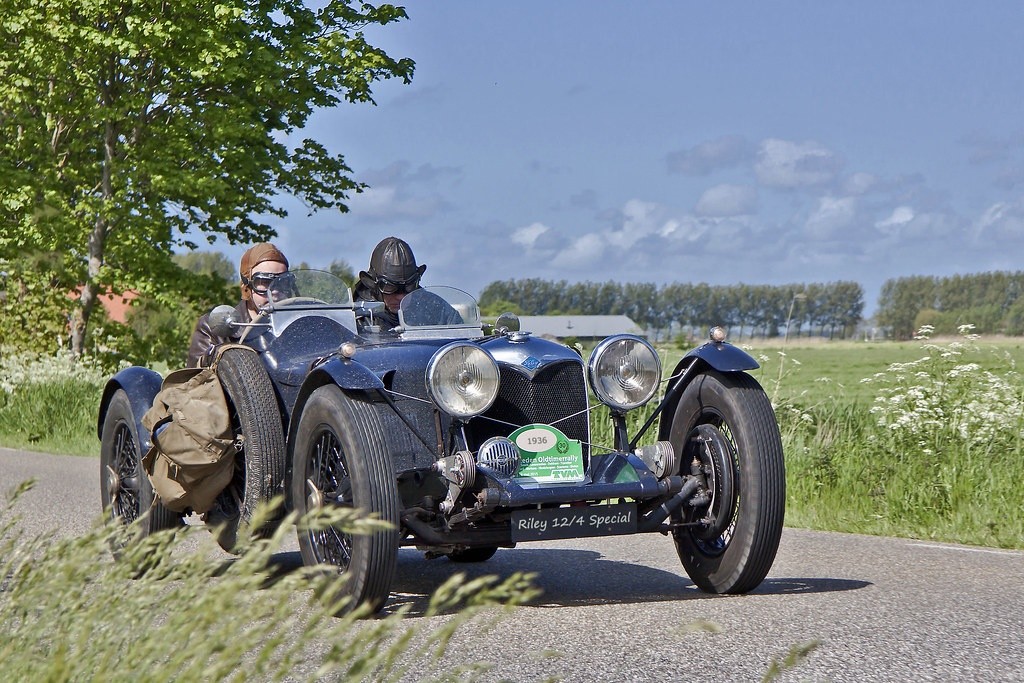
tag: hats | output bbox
[359,237,426,294]
[240,243,289,275]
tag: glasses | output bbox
[377,278,420,295]
[251,272,290,291]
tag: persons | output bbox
[186,243,289,368]
[344,236,463,335]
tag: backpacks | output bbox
[140,344,257,514]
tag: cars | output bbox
[98,270,786,619]
